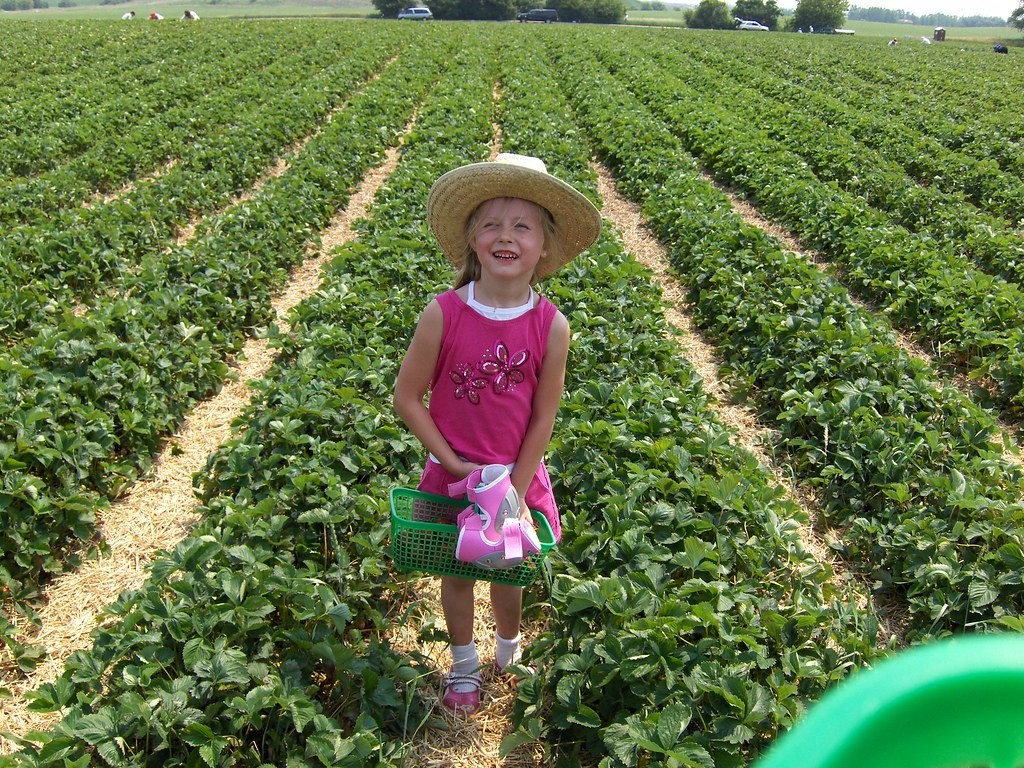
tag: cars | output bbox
[734,18,769,31]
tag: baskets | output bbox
[389,486,556,587]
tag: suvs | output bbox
[515,8,559,23]
[397,8,434,21]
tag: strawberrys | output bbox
[396,518,539,584]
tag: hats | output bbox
[426,153,602,285]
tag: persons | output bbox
[797,27,802,34]
[887,37,898,47]
[624,12,628,24]
[809,26,814,34]
[392,152,604,713]
[147,12,164,20]
[122,11,134,20]
[180,8,200,20]
[920,36,932,45]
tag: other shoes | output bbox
[494,658,537,690]
[443,667,483,717]
